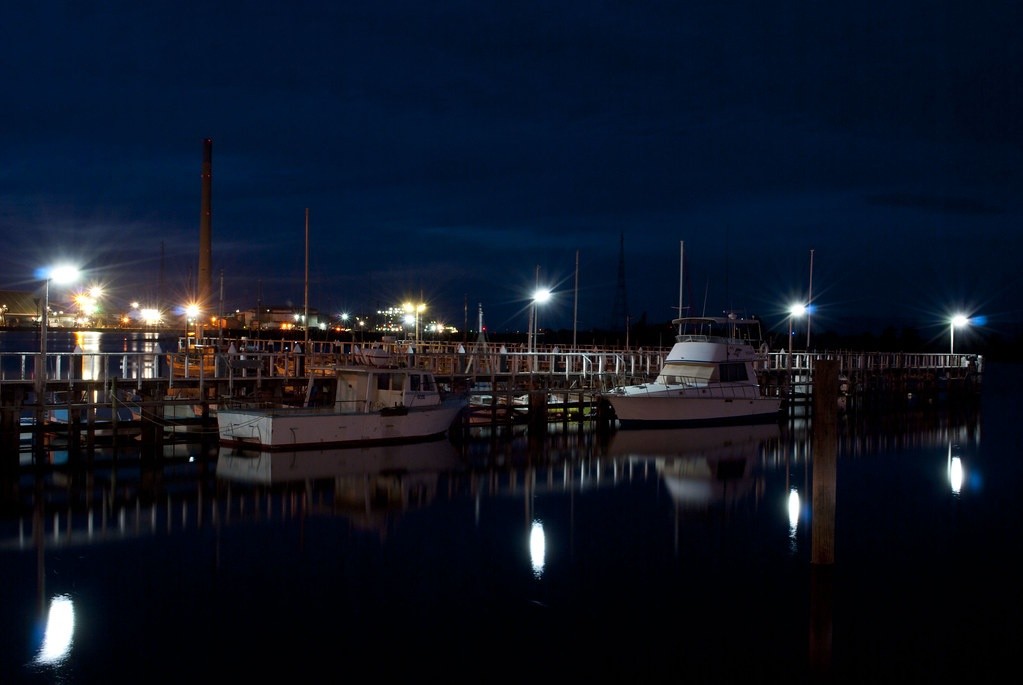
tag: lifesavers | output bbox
[758,342,768,358]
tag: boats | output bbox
[601,332,783,426]
[607,424,782,506]
[672,315,764,349]
[946,437,966,495]
[216,441,471,485]
[19,402,219,449]
[219,343,473,452]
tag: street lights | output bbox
[182,304,203,355]
[787,300,806,353]
[402,300,429,341]
[530,285,551,370]
[948,311,970,354]
[528,467,547,574]
[783,435,802,533]
